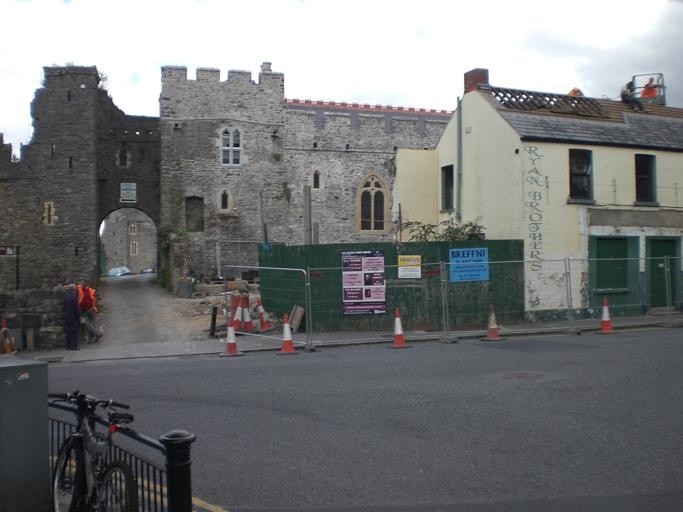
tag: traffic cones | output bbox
[221,293,273,335]
[215,316,246,359]
[479,301,511,342]
[383,307,414,350]
[593,296,620,333]
[270,312,301,356]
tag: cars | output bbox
[104,265,130,276]
[140,266,158,273]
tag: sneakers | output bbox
[87,333,102,344]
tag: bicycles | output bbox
[47,388,137,512]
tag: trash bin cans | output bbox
[5,313,39,351]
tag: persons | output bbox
[64,284,83,351]
[640,77,659,98]
[64,279,104,344]
[619,81,644,113]
